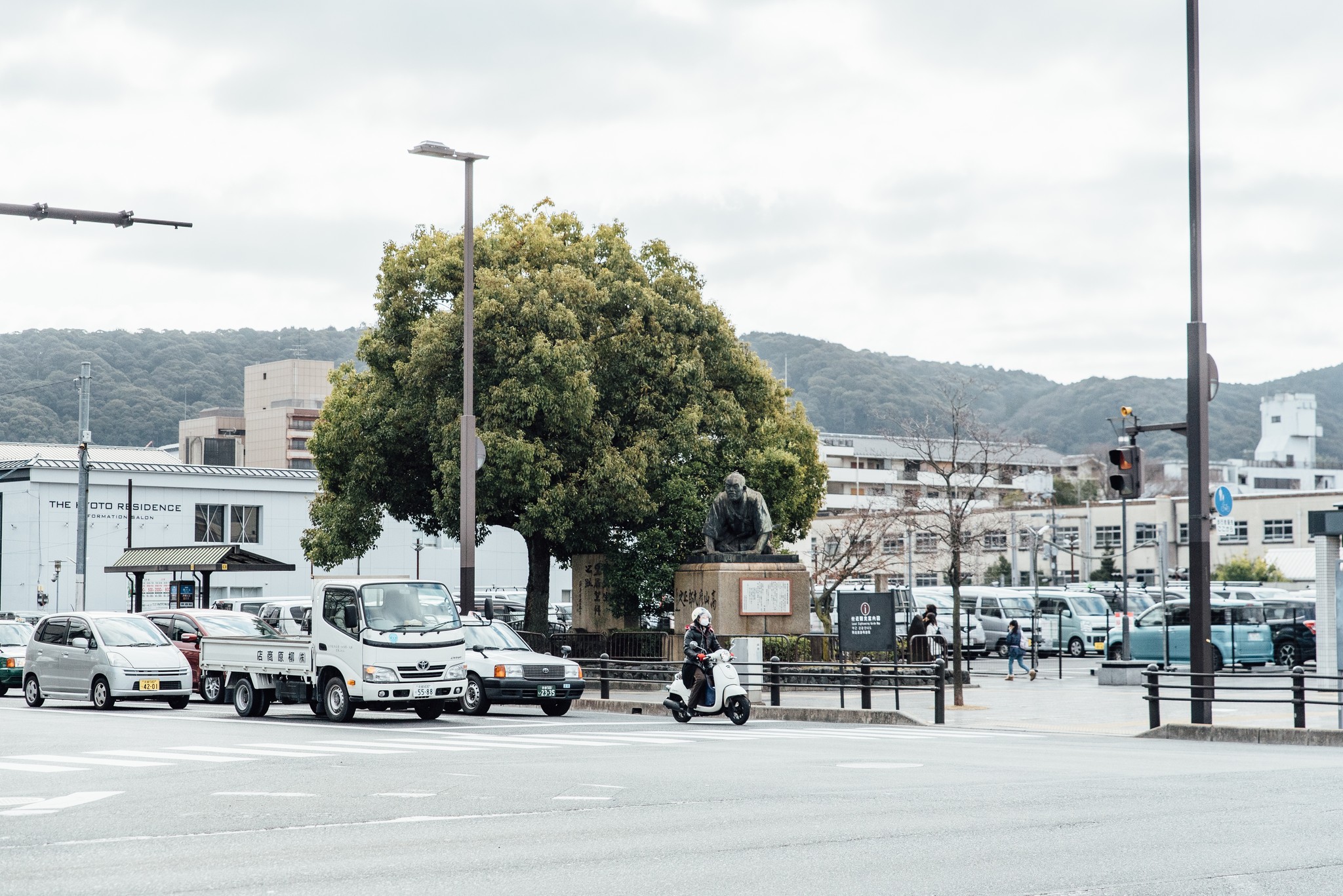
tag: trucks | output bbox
[200,579,467,724]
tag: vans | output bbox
[206,595,313,618]
[0,610,51,628]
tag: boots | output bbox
[1029,669,1036,681]
[1004,674,1014,681]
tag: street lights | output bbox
[405,140,490,614]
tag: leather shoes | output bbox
[687,707,695,717]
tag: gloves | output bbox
[697,653,706,661]
[730,652,734,656]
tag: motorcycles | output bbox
[663,637,751,727]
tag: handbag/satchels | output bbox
[704,675,715,705]
[1020,629,1028,650]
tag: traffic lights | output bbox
[1109,445,1145,500]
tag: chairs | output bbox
[328,610,341,626]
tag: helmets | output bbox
[692,607,712,623]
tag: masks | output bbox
[696,618,709,626]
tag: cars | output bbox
[0,617,37,697]
[409,611,587,716]
[135,609,283,705]
[256,586,573,634]
[21,610,194,711]
[811,580,1316,668]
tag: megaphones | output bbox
[79,443,85,450]
[1120,405,1133,417]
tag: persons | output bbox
[1005,620,1037,681]
[905,614,932,664]
[683,607,735,717]
[922,604,938,628]
[925,612,941,664]
[702,469,775,555]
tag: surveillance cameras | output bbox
[50,575,57,583]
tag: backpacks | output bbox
[929,624,944,644]
